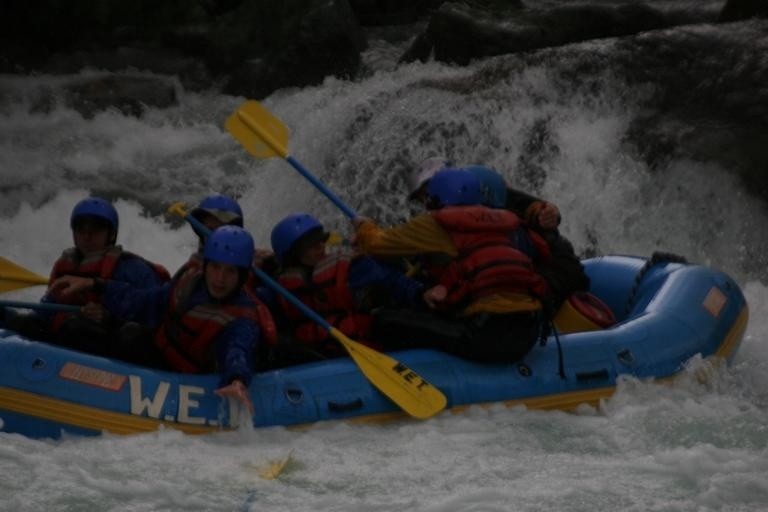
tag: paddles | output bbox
[223,99,429,292]
[0,254,49,297]
[169,200,448,422]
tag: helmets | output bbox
[424,166,488,206]
[200,225,255,270]
[269,211,325,262]
[461,163,509,209]
[198,194,243,216]
[70,197,120,232]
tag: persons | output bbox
[9,200,171,350]
[406,150,590,321]
[170,194,273,292]
[253,213,448,358]
[350,167,561,364]
[45,224,258,416]
